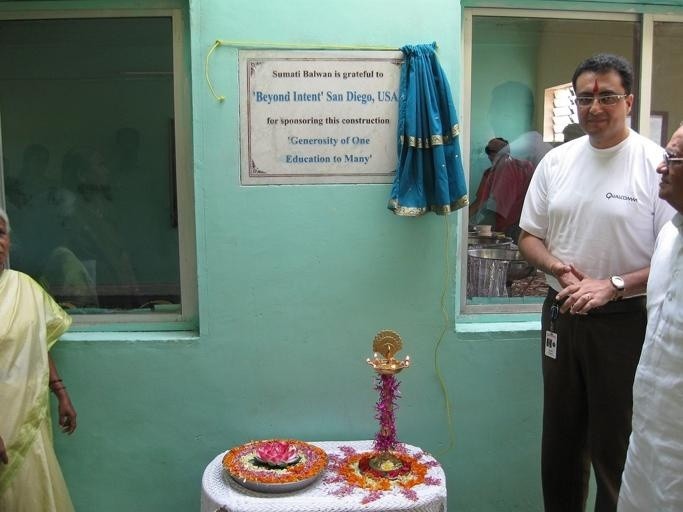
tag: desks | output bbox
[200,439,447,512]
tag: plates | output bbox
[222,439,330,493]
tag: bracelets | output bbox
[50,386,65,391]
[48,378,62,388]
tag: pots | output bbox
[467,230,539,297]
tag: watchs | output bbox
[608,271,624,301]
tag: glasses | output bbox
[661,149,682,165]
[573,93,627,108]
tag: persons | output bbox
[470,137,535,246]
[615,122,683,511]
[517,53,676,511]
[0,206,77,511]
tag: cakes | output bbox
[221,437,329,486]
